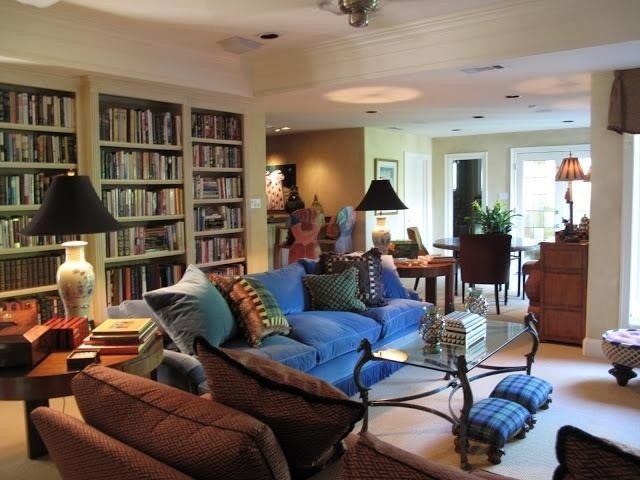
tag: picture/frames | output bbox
[372,157,399,216]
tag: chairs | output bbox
[408,227,459,290]
[323,204,358,256]
[458,231,512,313]
[521,257,538,300]
[287,208,327,267]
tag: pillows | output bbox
[552,420,640,478]
[139,264,238,356]
[70,363,291,478]
[301,270,368,315]
[317,248,389,307]
[297,253,410,300]
[207,272,292,348]
[195,336,366,470]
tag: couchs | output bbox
[109,246,440,406]
[27,340,640,480]
[523,255,542,315]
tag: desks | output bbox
[267,215,294,271]
[0,323,167,462]
[601,327,640,392]
[429,235,541,297]
[344,251,460,315]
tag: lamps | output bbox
[352,179,409,257]
[19,167,123,330]
[552,151,590,242]
[265,170,287,222]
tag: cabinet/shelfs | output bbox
[0,60,96,330]
[185,93,252,281]
[537,238,588,348]
[78,71,191,327]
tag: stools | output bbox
[446,392,532,470]
[494,369,552,427]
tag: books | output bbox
[106,222,185,258]
[191,112,242,140]
[106,260,186,307]
[1,253,65,292]
[1,90,82,248]
[192,143,242,168]
[100,108,183,180]
[192,174,241,199]
[2,293,64,326]
[208,263,244,276]
[194,204,241,231]
[196,235,244,264]
[102,188,184,218]
[78,317,160,356]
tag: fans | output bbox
[331,0,385,31]
